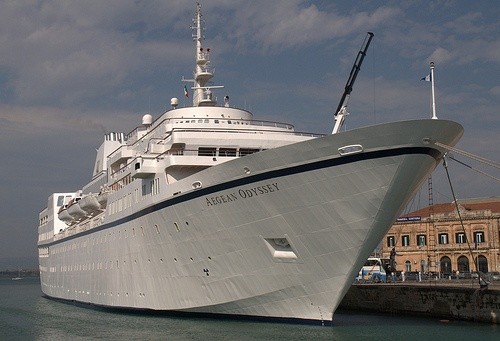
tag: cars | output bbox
[404,272,427,280]
[471,271,486,280]
[487,271,500,281]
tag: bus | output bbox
[355,258,396,283]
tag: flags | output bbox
[184,85,189,97]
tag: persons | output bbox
[224,95,230,104]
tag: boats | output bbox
[38,2,465,321]
